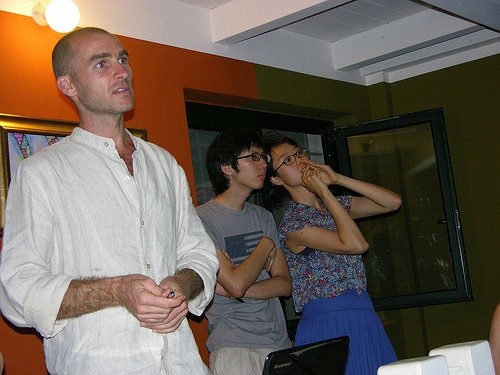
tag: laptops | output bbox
[262,335,351,375]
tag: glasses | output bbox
[273,148,307,174]
[235,152,271,163]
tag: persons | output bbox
[195,125,293,375]
[0,27,220,375]
[262,132,403,375]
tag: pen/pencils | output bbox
[166,292,174,298]
